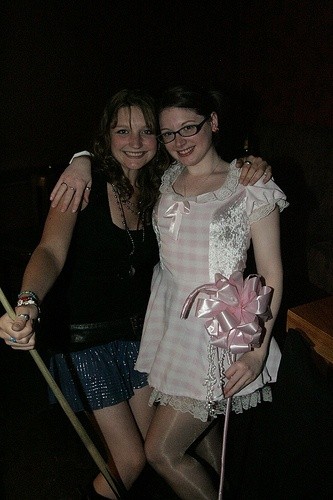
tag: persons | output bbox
[0,87,274,500]
[49,87,289,500]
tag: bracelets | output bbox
[17,290,41,314]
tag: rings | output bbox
[61,181,68,186]
[244,160,252,165]
[251,167,257,171]
[18,314,28,319]
[85,186,90,190]
[69,186,75,190]
[10,337,17,343]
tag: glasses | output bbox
[156,118,210,144]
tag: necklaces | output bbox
[183,159,222,196]
[110,182,146,276]
